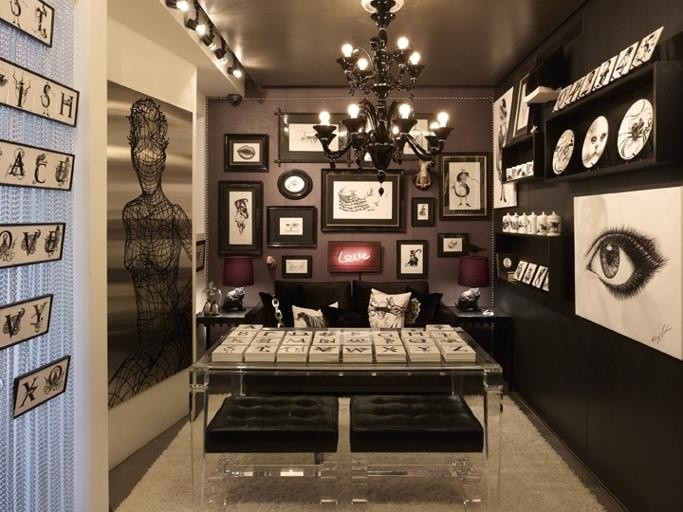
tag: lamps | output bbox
[221,256,255,312]
[314,0,455,196]
[454,256,492,312]
[165,0,254,81]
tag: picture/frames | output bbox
[272,110,436,169]
[435,231,471,259]
[221,132,272,174]
[317,168,410,234]
[395,239,431,279]
[215,178,266,257]
[326,239,384,274]
[437,149,490,222]
[280,253,314,279]
[265,204,319,251]
[409,196,438,228]
[276,169,312,202]
[1,0,84,422]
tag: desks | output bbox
[448,304,513,389]
[197,305,255,351]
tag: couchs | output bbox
[247,279,458,325]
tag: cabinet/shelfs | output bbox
[494,232,563,302]
[543,60,681,184]
[188,326,504,510]
[500,132,543,184]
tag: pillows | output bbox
[367,287,410,327]
[259,292,307,327]
[290,300,339,327]
[319,305,369,328]
[400,286,443,326]
